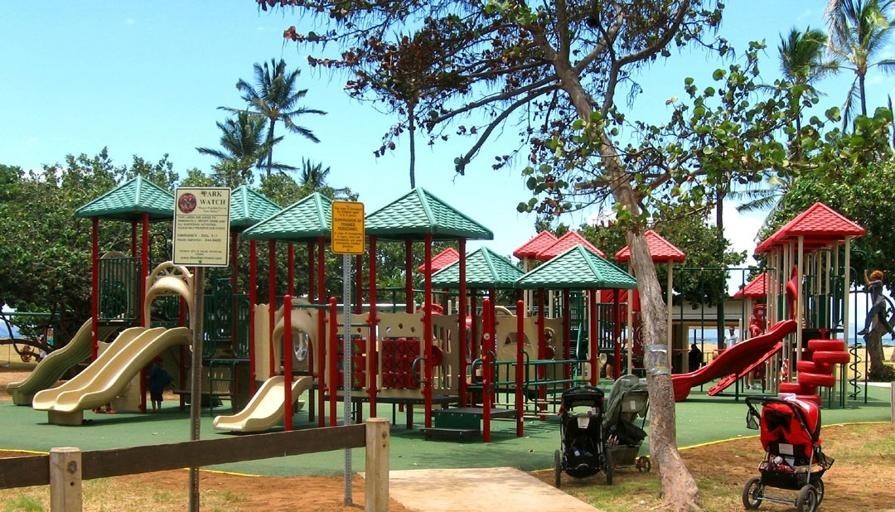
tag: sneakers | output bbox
[892,333,895,340]
[857,328,869,335]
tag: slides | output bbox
[6,317,92,395]
[33,326,191,413]
[671,319,797,401]
[214,375,312,432]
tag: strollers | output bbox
[742,395,834,511]
[554,375,651,488]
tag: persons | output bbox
[724,326,740,349]
[689,344,703,371]
[36,333,51,360]
[147,363,172,413]
[858,268,895,342]
[604,352,625,380]
[150,302,162,321]
